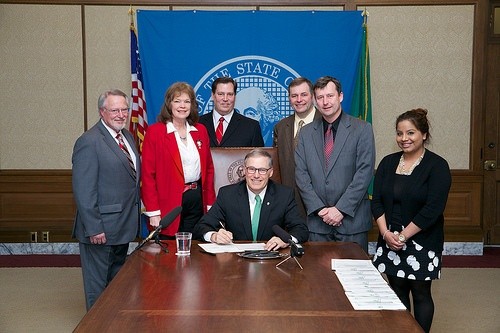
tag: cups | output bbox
[175,232,192,254]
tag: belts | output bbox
[182,182,197,193]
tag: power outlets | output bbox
[30,232,37,242]
[42,232,49,242]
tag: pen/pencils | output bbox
[219,221,235,243]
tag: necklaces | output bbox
[180,137,187,139]
[399,149,425,175]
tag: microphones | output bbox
[135,205,182,250]
[271,225,299,254]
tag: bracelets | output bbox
[383,230,390,239]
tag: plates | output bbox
[236,251,289,258]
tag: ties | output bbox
[324,124,334,169]
[293,120,304,149]
[251,194,262,241]
[215,117,225,145]
[115,134,137,179]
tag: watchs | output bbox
[398,233,407,243]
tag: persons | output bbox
[295,77,376,254]
[370,108,452,333]
[193,151,309,251]
[72,89,141,311]
[199,78,264,148]
[271,78,322,222]
[139,82,217,240]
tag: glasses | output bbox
[245,165,271,175]
[104,106,130,114]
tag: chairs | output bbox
[210,145,283,199]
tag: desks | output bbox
[72,240,424,333]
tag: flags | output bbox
[350,22,374,200]
[129,23,148,155]
